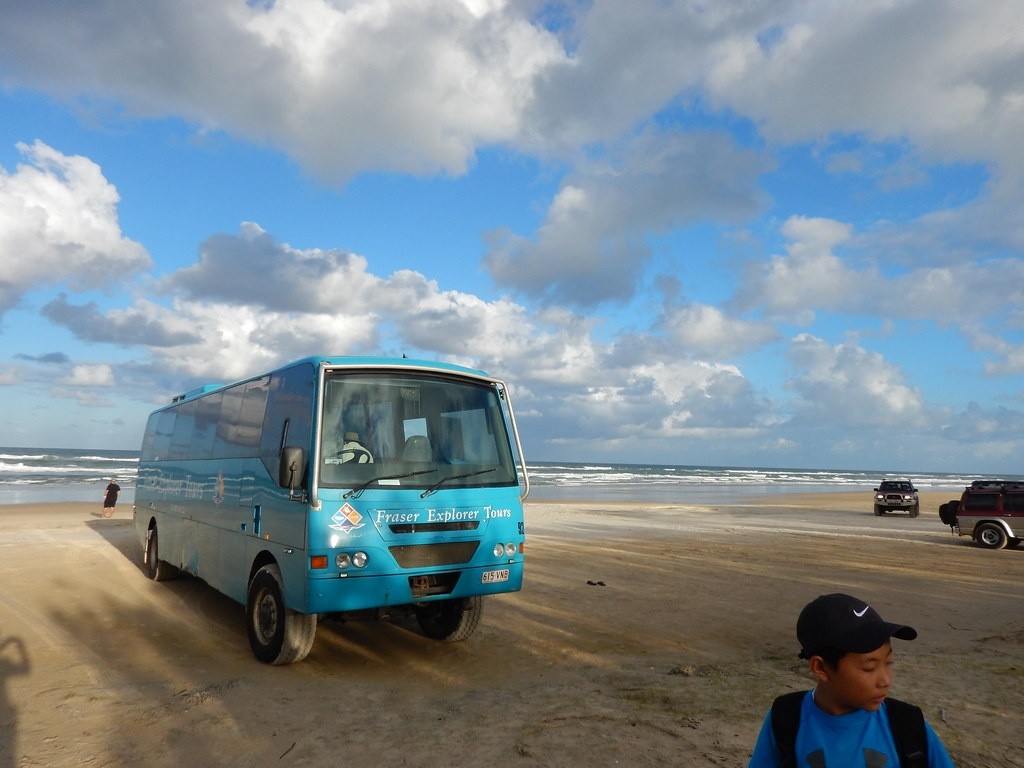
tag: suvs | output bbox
[872,477,920,518]
[938,479,1024,549]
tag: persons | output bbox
[335,432,373,463]
[102,479,121,518]
[748,593,955,768]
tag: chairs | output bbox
[401,435,433,464]
[901,485,907,491]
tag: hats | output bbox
[342,431,365,444]
[797,591,917,660]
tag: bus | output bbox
[131,355,531,667]
